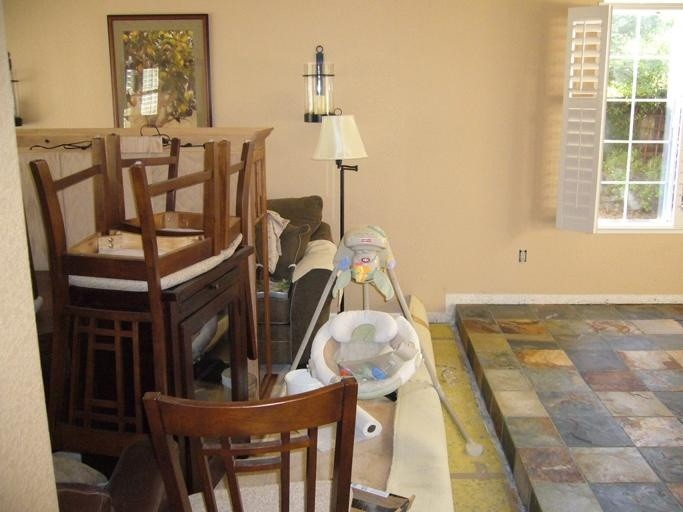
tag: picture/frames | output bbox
[106,12,213,128]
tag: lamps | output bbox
[312,115,369,312]
[302,44,343,124]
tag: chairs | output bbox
[142,378,360,510]
[255,220,337,364]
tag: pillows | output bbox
[267,194,324,282]
